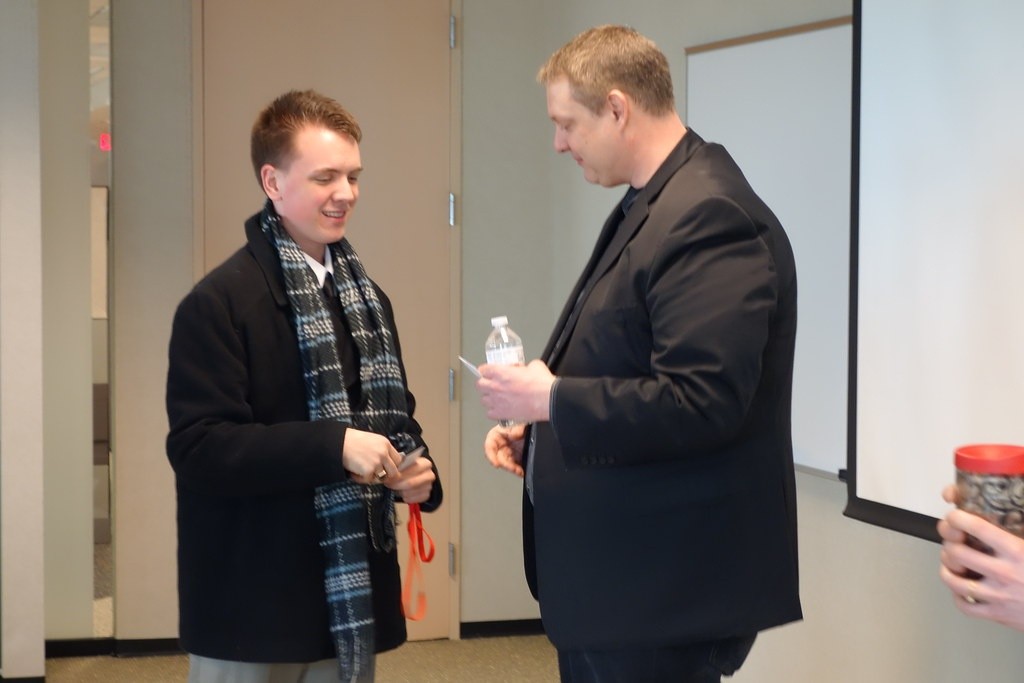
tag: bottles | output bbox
[485,315,526,430]
[954,444,1024,605]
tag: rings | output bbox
[374,470,386,480]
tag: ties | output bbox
[322,276,341,317]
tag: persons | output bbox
[166,88,444,683]
[936,485,1024,634]
[476,24,804,683]
